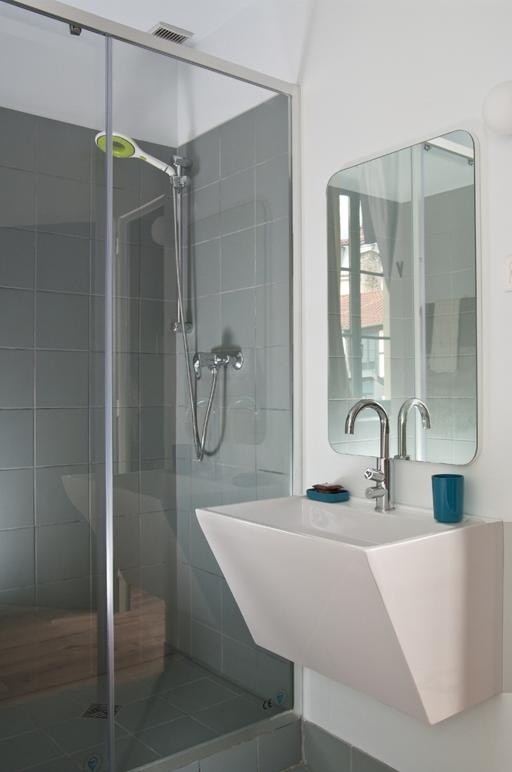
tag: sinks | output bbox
[196,492,506,724]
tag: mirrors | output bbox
[327,132,479,466]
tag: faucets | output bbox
[345,399,395,511]
[395,399,431,461]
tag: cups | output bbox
[431,473,464,524]
